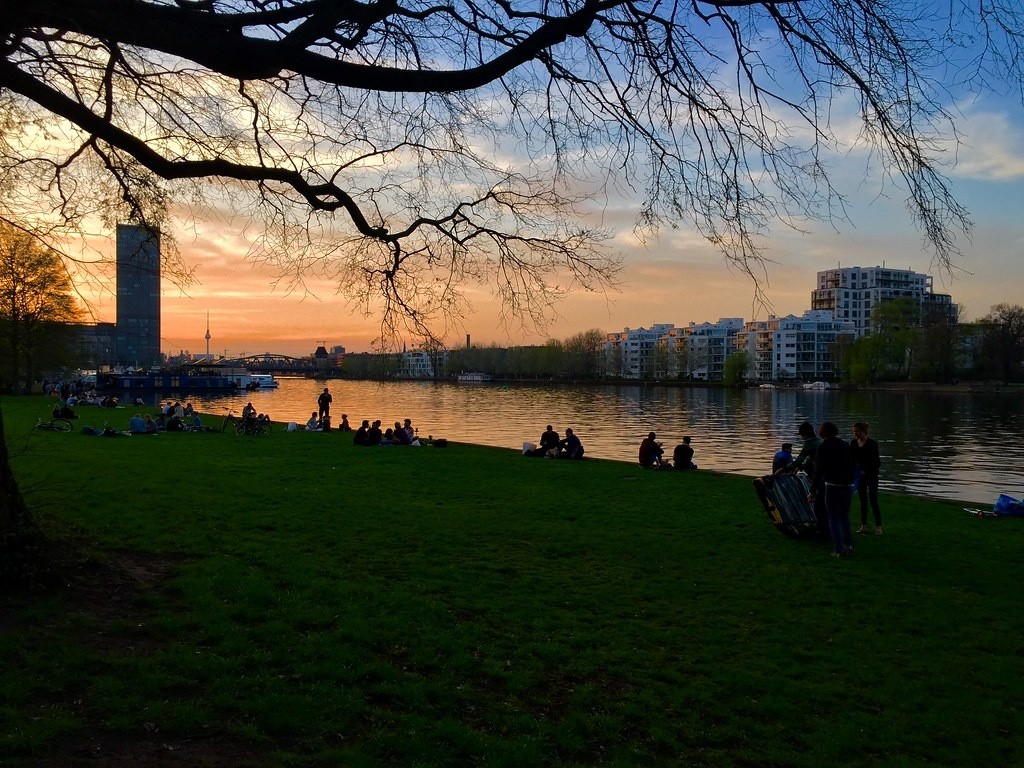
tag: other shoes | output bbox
[875,526,882,536]
[856,527,868,534]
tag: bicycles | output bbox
[221,406,246,437]
[35,417,73,434]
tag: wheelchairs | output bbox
[243,415,272,437]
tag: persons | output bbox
[243,403,269,427]
[524,425,580,458]
[639,433,698,471]
[353,419,414,445]
[772,422,884,557]
[305,388,349,432]
[43,373,201,434]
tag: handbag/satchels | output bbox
[288,422,297,430]
[522,443,536,454]
[412,438,421,446]
